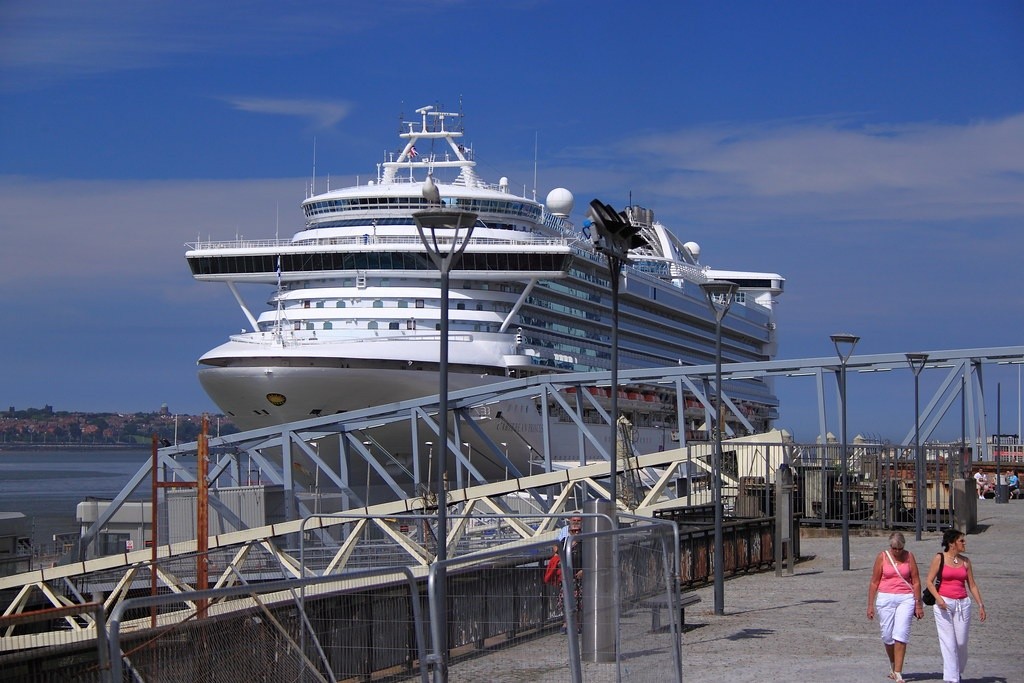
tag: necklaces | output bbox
[889,550,898,566]
[946,553,958,564]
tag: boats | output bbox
[587,387,609,409]
[674,398,702,420]
[533,388,568,406]
[698,401,716,421]
[642,394,661,416]
[565,387,590,409]
[606,388,630,412]
[627,392,647,411]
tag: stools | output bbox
[639,593,702,631]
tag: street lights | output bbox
[699,280,741,617]
[362,441,372,543]
[499,441,509,480]
[585,195,652,659]
[309,441,320,514]
[904,351,931,540]
[525,444,534,513]
[408,208,479,683]
[831,332,862,571]
[423,441,434,542]
[461,441,471,487]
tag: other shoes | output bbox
[577,626,582,634]
[887,666,906,683]
[978,495,986,500]
[560,626,567,635]
[1010,494,1014,499]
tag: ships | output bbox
[180,102,789,518]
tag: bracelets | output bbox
[869,603,873,605]
[915,600,921,602]
[979,603,984,608]
[916,607,922,609]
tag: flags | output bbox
[409,145,419,159]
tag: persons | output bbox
[552,511,584,635]
[866,532,924,683]
[973,467,1020,500]
[926,529,986,683]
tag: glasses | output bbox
[889,544,904,551]
[568,528,581,534]
[958,538,965,542]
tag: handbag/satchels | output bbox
[922,552,944,606]
